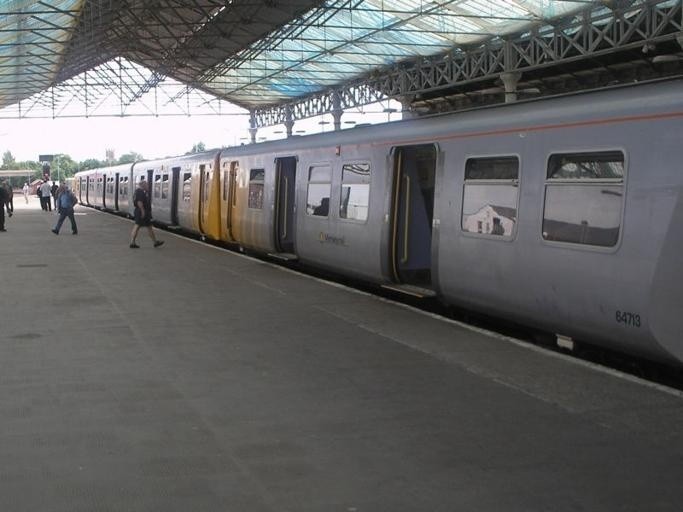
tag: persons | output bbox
[36,182,47,210]
[129,179,165,249]
[49,181,58,211]
[0,178,14,232]
[38,178,51,212]
[55,182,65,199]
[21,182,29,204]
[50,184,78,236]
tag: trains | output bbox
[71,69,683,385]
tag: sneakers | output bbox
[154,240,164,248]
[52,229,58,234]
[129,243,140,248]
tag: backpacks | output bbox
[60,192,74,209]
